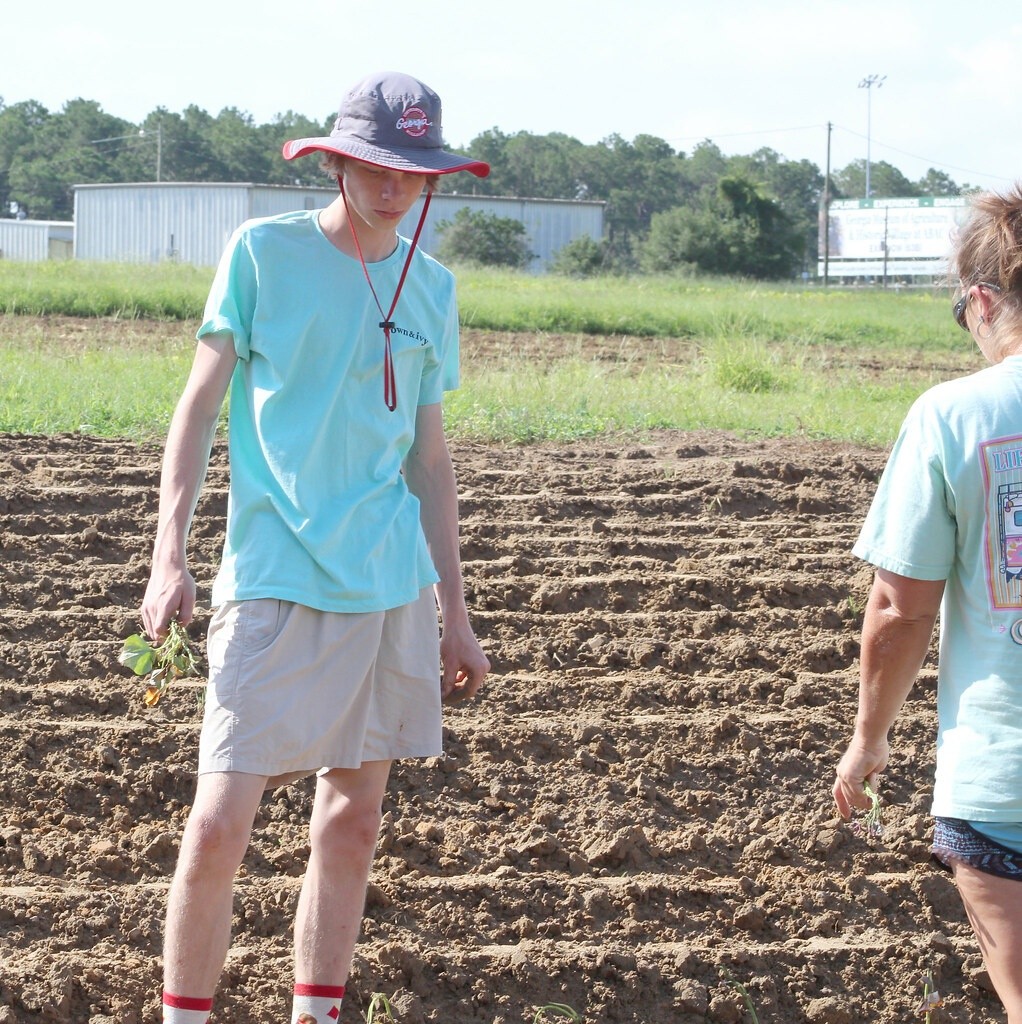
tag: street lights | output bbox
[859,72,886,200]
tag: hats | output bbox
[283,71,490,179]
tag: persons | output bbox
[138,70,490,1024]
[831,182,1022,1024]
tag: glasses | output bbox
[952,282,1001,331]
